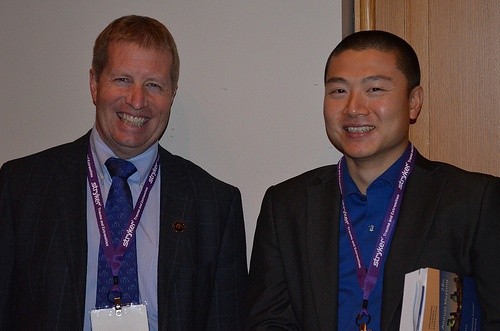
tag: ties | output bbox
[93,156,140,309]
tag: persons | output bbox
[246,31,500,330]
[0,16,248,331]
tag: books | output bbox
[399,267,484,331]
